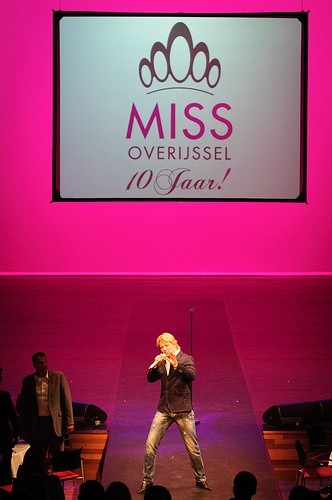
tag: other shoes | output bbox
[137,483,154,494]
[196,480,212,492]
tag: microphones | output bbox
[148,354,166,370]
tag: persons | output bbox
[21,352,76,458]
[0,368,20,485]
[0,446,172,500]
[137,333,213,493]
[228,470,257,500]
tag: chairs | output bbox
[48,447,84,494]
[295,440,332,495]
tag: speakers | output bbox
[320,400,332,425]
[72,402,107,427]
[263,400,323,429]
